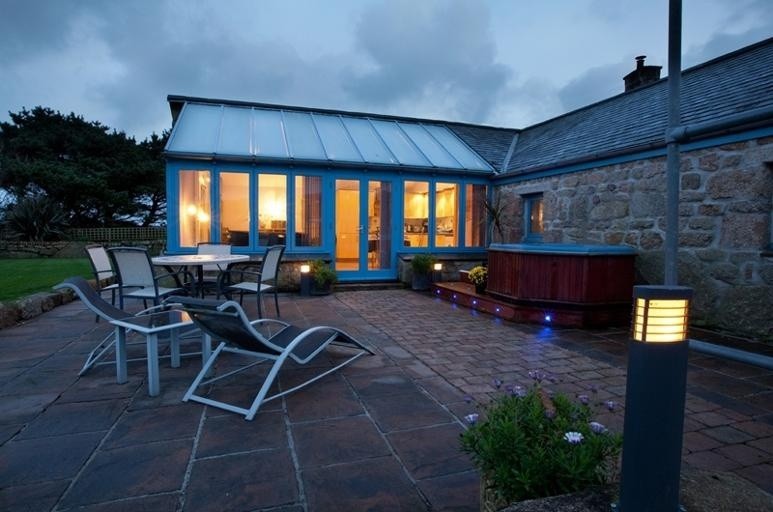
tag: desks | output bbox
[108,309,211,396]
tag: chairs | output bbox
[84,242,286,325]
[161,295,375,421]
[54,279,240,378]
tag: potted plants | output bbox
[407,254,438,290]
[304,258,338,295]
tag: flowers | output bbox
[456,368,621,493]
[468,266,488,284]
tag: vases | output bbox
[474,283,486,294]
[481,473,612,511]
